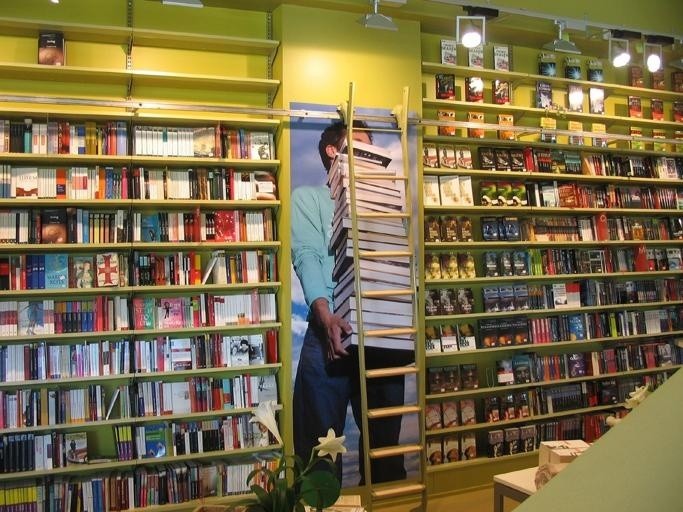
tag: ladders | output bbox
[345,80,428,512]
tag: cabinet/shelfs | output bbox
[2,1,299,511]
[406,11,682,500]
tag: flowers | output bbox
[225,398,349,512]
[601,378,656,434]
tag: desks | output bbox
[488,463,544,512]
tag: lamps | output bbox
[455,5,499,48]
[358,1,399,31]
[642,35,669,75]
[542,17,586,55]
[606,28,642,67]
